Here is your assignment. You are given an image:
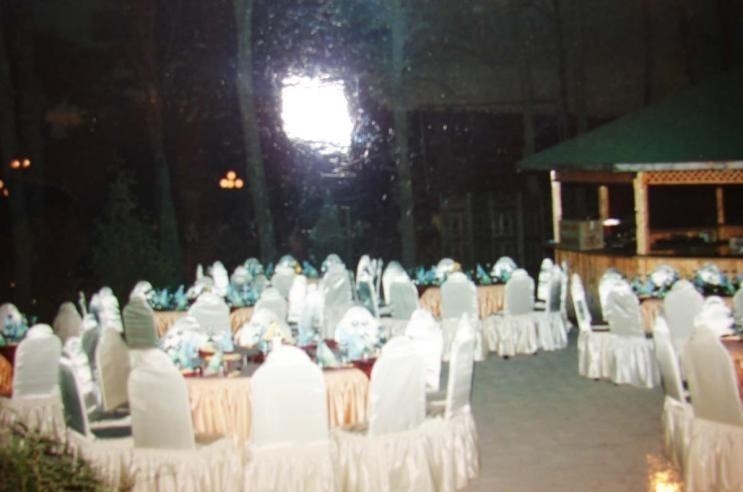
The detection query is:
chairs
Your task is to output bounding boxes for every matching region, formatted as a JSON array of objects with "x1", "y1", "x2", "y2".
[
  {"x1": 0, "y1": 304, "x2": 64, "y2": 443},
  {"x1": 56, "y1": 277, "x2": 482, "y2": 492},
  {"x1": 653, "y1": 277, "x2": 742, "y2": 492},
  {"x1": 570, "y1": 262, "x2": 743, "y2": 389},
  {"x1": 131, "y1": 254, "x2": 574, "y2": 360}
]
